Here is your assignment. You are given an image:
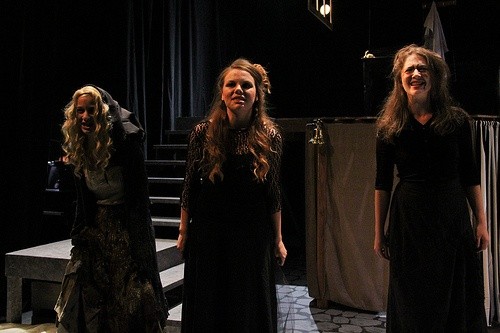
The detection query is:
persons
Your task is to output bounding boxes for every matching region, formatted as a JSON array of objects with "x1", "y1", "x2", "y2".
[
  {"x1": 177, "y1": 58, "x2": 288, "y2": 333},
  {"x1": 374, "y1": 44, "x2": 491, "y2": 332},
  {"x1": 53, "y1": 84, "x2": 169, "y2": 333}
]
